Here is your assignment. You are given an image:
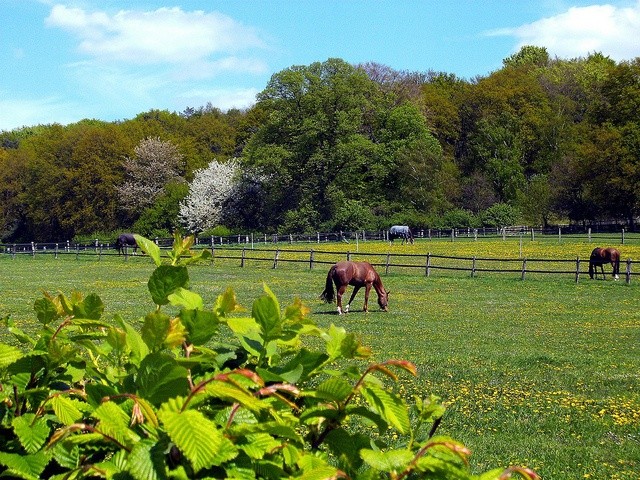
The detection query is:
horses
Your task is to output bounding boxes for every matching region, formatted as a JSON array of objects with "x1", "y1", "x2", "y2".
[
  {"x1": 117, "y1": 233, "x2": 138, "y2": 254},
  {"x1": 318, "y1": 260, "x2": 391, "y2": 315},
  {"x1": 588, "y1": 246, "x2": 620, "y2": 282},
  {"x1": 387, "y1": 225, "x2": 415, "y2": 246}
]
